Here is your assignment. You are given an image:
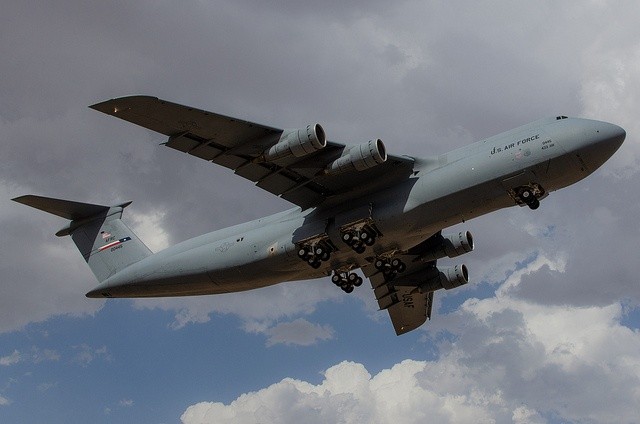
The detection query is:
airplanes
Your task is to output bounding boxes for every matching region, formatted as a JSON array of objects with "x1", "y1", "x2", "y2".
[{"x1": 10, "y1": 94, "x2": 626, "y2": 336}]
